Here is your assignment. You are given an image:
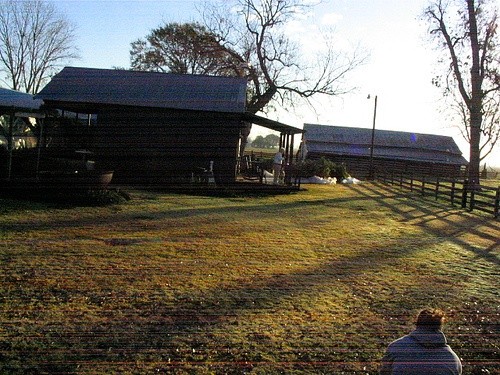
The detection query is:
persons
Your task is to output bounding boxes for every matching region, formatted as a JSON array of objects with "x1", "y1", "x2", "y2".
[
  {"x1": 378, "y1": 308, "x2": 463, "y2": 375},
  {"x1": 272, "y1": 148, "x2": 285, "y2": 186}
]
